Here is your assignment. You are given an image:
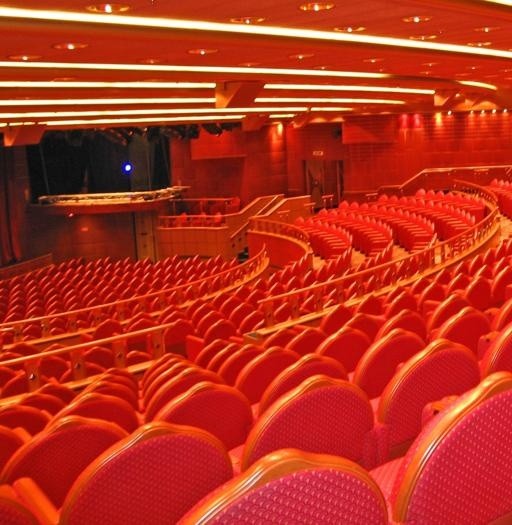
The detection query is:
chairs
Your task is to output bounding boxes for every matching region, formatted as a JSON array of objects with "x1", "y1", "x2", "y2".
[{"x1": 1, "y1": 179, "x2": 512, "y2": 525}]
